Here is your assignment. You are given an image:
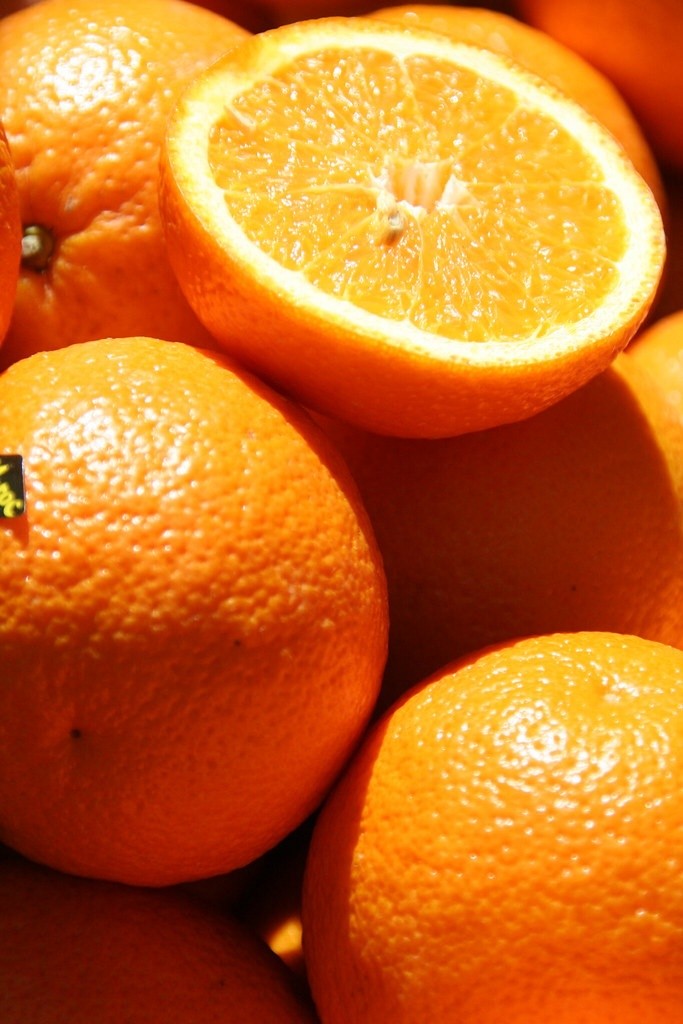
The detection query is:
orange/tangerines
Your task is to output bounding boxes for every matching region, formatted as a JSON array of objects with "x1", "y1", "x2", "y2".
[{"x1": 0, "y1": 0, "x2": 683, "y2": 1024}]
